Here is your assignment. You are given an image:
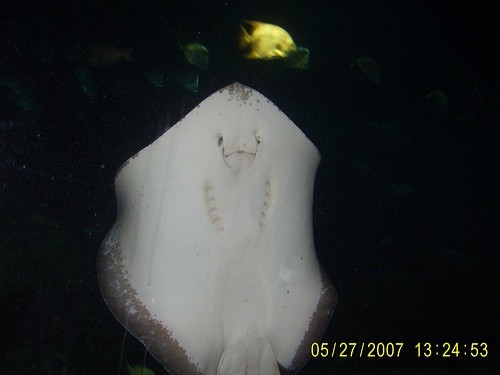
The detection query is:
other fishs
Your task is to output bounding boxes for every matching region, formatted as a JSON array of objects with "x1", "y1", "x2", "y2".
[
  {"x1": 235, "y1": 18, "x2": 299, "y2": 61},
  {"x1": 78, "y1": 41, "x2": 133, "y2": 70},
  {"x1": 95, "y1": 77, "x2": 339, "y2": 375},
  {"x1": 284, "y1": 46, "x2": 310, "y2": 70},
  {"x1": 177, "y1": 40, "x2": 210, "y2": 73}
]
